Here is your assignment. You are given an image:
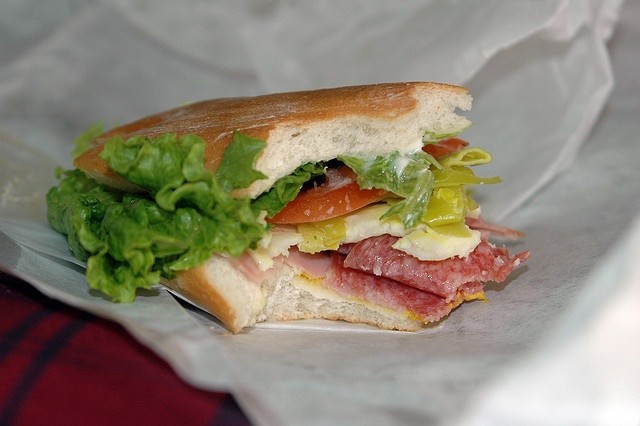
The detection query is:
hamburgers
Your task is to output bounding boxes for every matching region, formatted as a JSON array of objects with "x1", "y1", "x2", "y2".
[{"x1": 45, "y1": 80, "x2": 533, "y2": 337}]
